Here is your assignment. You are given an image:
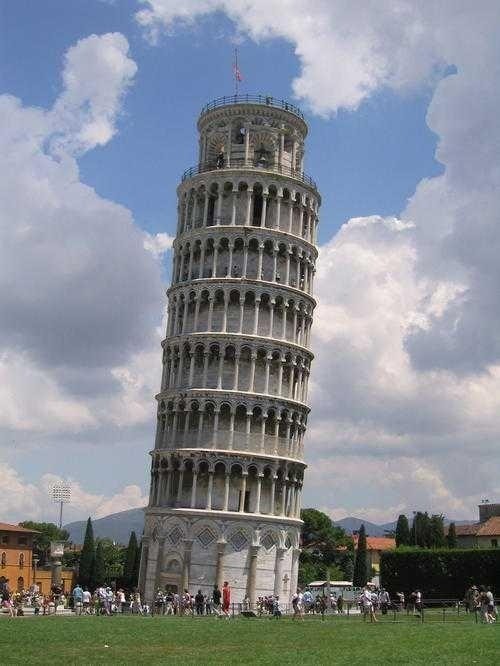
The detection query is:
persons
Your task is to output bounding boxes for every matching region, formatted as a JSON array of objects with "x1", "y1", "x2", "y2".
[
  {"x1": 360, "y1": 585, "x2": 422, "y2": 622},
  {"x1": 464, "y1": 583, "x2": 497, "y2": 624},
  {"x1": 291, "y1": 588, "x2": 343, "y2": 622},
  {"x1": 244, "y1": 594, "x2": 279, "y2": 615},
  {"x1": 1, "y1": 582, "x2": 230, "y2": 617}
]
